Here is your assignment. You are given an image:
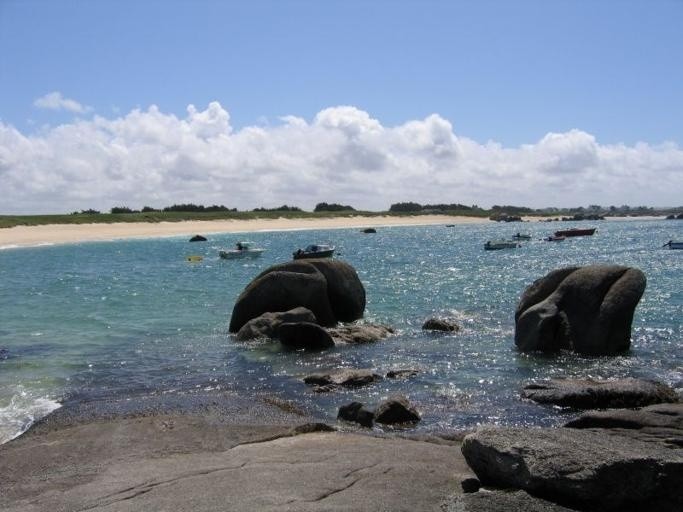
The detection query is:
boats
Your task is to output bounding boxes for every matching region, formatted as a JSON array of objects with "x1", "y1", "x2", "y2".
[
  {"x1": 218, "y1": 242, "x2": 265, "y2": 260},
  {"x1": 511, "y1": 232, "x2": 532, "y2": 240},
  {"x1": 554, "y1": 228, "x2": 595, "y2": 237},
  {"x1": 483, "y1": 241, "x2": 521, "y2": 250},
  {"x1": 445, "y1": 225, "x2": 455, "y2": 227},
  {"x1": 662, "y1": 240, "x2": 683, "y2": 250},
  {"x1": 291, "y1": 243, "x2": 335, "y2": 258},
  {"x1": 538, "y1": 236, "x2": 565, "y2": 242}
]
